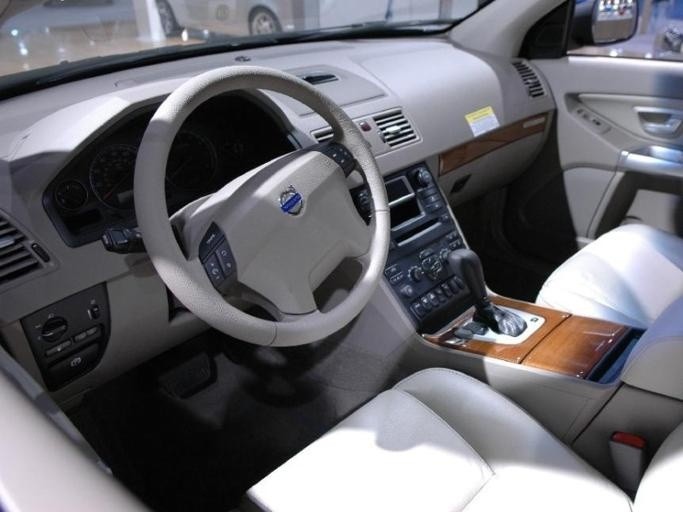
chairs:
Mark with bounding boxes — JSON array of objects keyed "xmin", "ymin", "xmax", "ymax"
[
  {"xmin": 532, "ymin": 222, "xmax": 683, "ymax": 398},
  {"xmin": 243, "ymin": 364, "xmax": 683, "ymax": 512}
]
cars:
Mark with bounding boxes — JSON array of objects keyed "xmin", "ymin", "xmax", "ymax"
[{"xmin": 158, "ymin": 2, "xmax": 296, "ymax": 44}]
